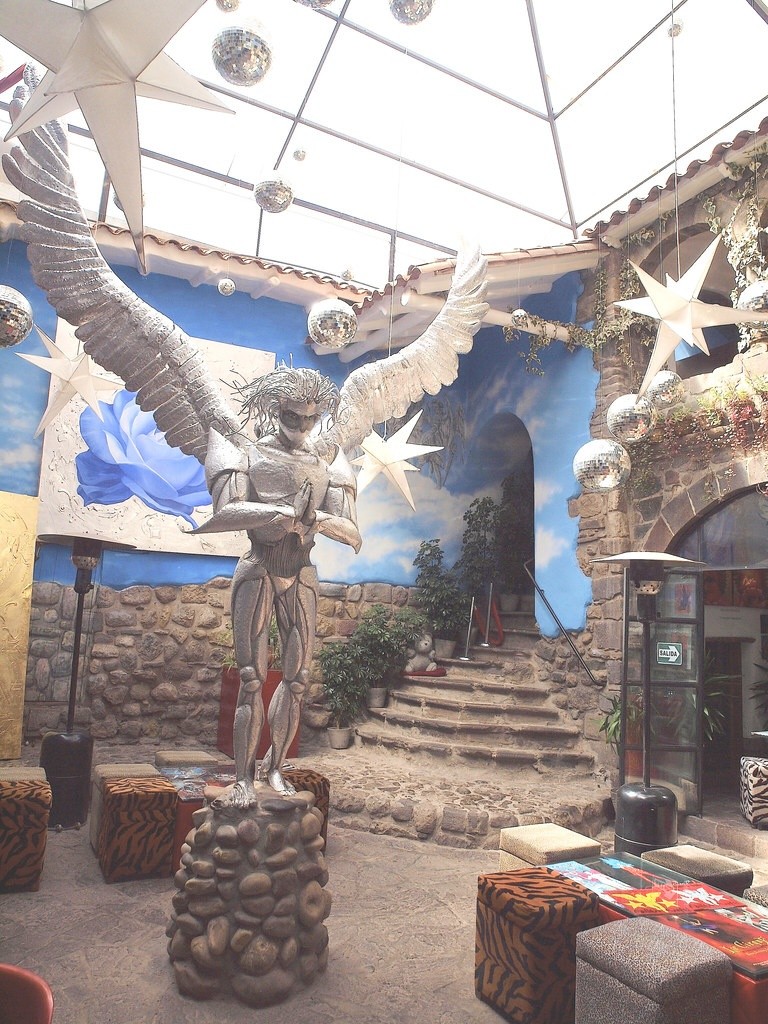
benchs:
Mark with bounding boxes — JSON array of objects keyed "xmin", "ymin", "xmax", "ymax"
[{"xmin": 545, "ymin": 850, "xmax": 768, "ymax": 1024}]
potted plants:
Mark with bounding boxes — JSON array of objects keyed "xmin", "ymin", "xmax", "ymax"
[
  {"xmin": 599, "ymin": 685, "xmax": 663, "ymax": 776},
  {"xmin": 663, "ymin": 647, "xmax": 744, "ymax": 779},
  {"xmin": 319, "ymin": 640, "xmax": 374, "ymax": 749},
  {"xmin": 217, "ymin": 605, "xmax": 300, "ymax": 760},
  {"xmin": 349, "ymin": 602, "xmax": 429, "ymax": 708},
  {"xmin": 451, "ymin": 496, "xmax": 498, "ymax": 645},
  {"xmin": 413, "ymin": 538, "xmax": 468, "ymax": 658},
  {"xmin": 498, "ymin": 470, "xmax": 534, "ymax": 613}
]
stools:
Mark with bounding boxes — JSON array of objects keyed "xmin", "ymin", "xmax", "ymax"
[
  {"xmin": 0, "ymin": 780, "xmax": 52, "ymax": 892},
  {"xmin": 739, "ymin": 757, "xmax": 768, "ymax": 831},
  {"xmin": 88, "ymin": 764, "xmax": 161, "ymax": 858},
  {"xmin": 98, "ymin": 777, "xmax": 178, "ymax": 884},
  {"xmin": 742, "ymin": 884, "xmax": 768, "ymax": 908},
  {"xmin": 574, "ymin": 916, "xmax": 733, "ymax": 1024},
  {"xmin": 0, "ymin": 767, "xmax": 46, "ymax": 780},
  {"xmin": 641, "ymin": 845, "xmax": 754, "ymax": 898},
  {"xmin": 155, "ymin": 751, "xmax": 330, "ymax": 878},
  {"xmin": 474, "ymin": 864, "xmax": 600, "ymax": 1024},
  {"xmin": 498, "ymin": 822, "xmax": 601, "ymax": 872}
]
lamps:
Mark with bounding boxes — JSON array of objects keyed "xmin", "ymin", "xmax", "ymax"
[
  {"xmin": 37, "ymin": 525, "xmax": 137, "ymax": 833},
  {"xmin": 589, "ymin": 552, "xmax": 708, "ymax": 857}
]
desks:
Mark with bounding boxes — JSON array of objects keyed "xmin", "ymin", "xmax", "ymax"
[{"xmin": 750, "ymin": 731, "xmax": 768, "ymax": 736}]
